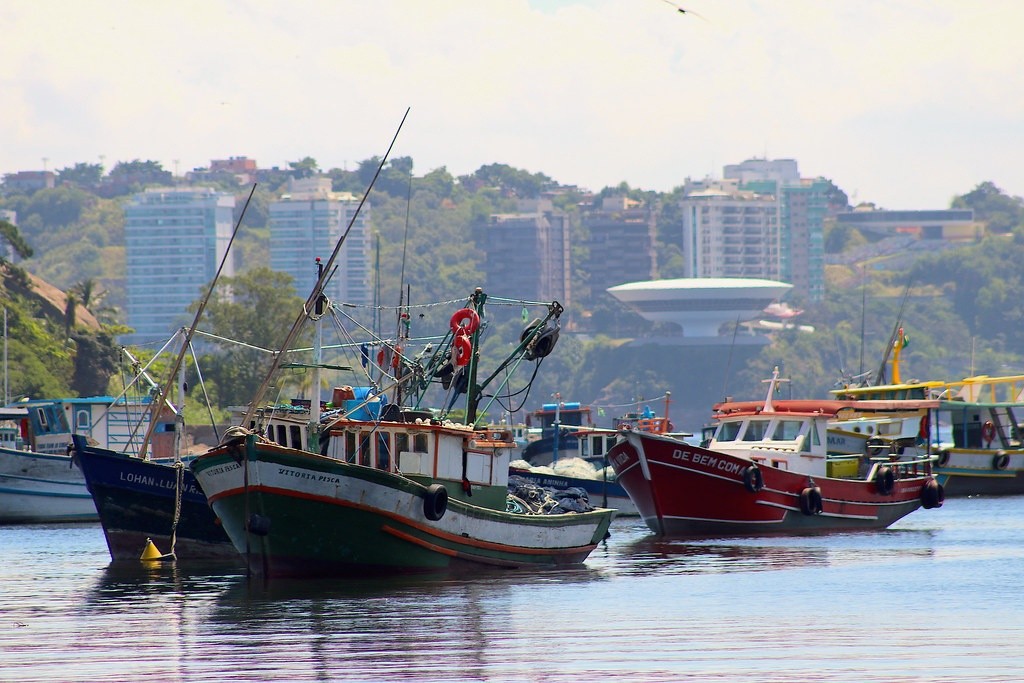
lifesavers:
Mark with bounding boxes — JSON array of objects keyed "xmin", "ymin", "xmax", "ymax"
[
  {"xmin": 376, "ymin": 345, "xmax": 401, "ymax": 368},
  {"xmin": 449, "ymin": 308, "xmax": 479, "ymax": 335},
  {"xmin": 669, "ymin": 423, "xmax": 673, "ymax": 431},
  {"xmin": 933, "ymin": 448, "xmax": 950, "ymax": 468},
  {"xmin": 801, "ymin": 487, "xmax": 823, "ymax": 516},
  {"xmin": 982, "ymin": 422, "xmax": 996, "ymax": 441},
  {"xmin": 865, "ymin": 435, "xmax": 884, "ymax": 456},
  {"xmin": 744, "ymin": 466, "xmax": 764, "ymax": 493},
  {"xmin": 423, "ymin": 483, "xmax": 448, "ymax": 521},
  {"xmin": 877, "ymin": 467, "xmax": 894, "ymax": 495},
  {"xmin": 451, "ymin": 336, "xmax": 472, "ymax": 366},
  {"xmin": 993, "ymin": 451, "xmax": 1010, "ymax": 470},
  {"xmin": 920, "ymin": 478, "xmax": 944, "ymax": 510},
  {"xmin": 919, "ymin": 415, "xmax": 928, "ymax": 440}
]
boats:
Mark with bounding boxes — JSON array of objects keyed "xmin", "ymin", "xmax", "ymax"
[
  {"xmin": 65, "ymin": 183, "xmax": 341, "ymax": 563},
  {"xmin": 0, "ymin": 397, "xmax": 200, "ymax": 525},
  {"xmin": 186, "ymin": 105, "xmax": 619, "ymax": 580},
  {"xmin": 506, "ymin": 392, "xmax": 694, "ymax": 518},
  {"xmin": 607, "ymin": 364, "xmax": 946, "ymax": 538},
  {"xmin": 826, "ymin": 266, "xmax": 1024, "ymax": 498}
]
